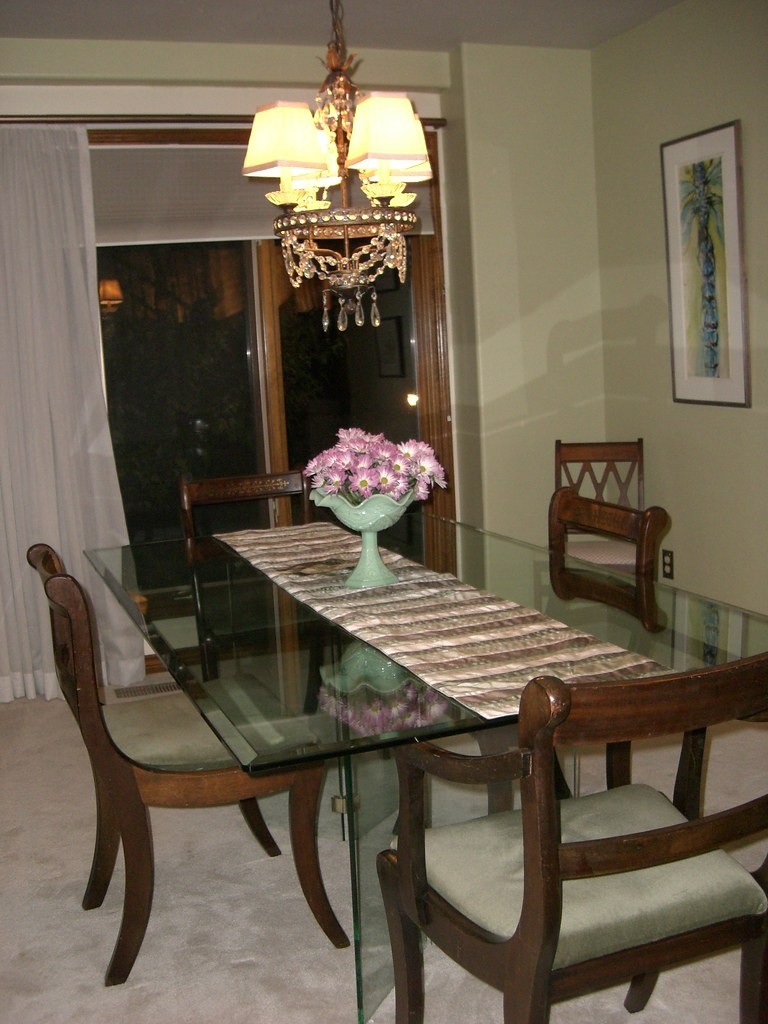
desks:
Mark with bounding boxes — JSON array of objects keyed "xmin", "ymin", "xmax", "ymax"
[{"xmin": 83, "ymin": 508, "xmax": 768, "ymax": 1024}]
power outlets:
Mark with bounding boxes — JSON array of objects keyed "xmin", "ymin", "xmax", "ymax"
[{"xmin": 662, "ymin": 549, "xmax": 674, "ymax": 579}]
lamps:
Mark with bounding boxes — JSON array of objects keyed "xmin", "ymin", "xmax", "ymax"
[
  {"xmin": 98, "ymin": 276, "xmax": 124, "ymax": 313},
  {"xmin": 239, "ymin": 0, "xmax": 433, "ymax": 332}
]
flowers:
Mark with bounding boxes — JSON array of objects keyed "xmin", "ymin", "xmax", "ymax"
[
  {"xmin": 318, "ymin": 688, "xmax": 450, "ymax": 736},
  {"xmin": 302, "ymin": 425, "xmax": 447, "ymax": 505}
]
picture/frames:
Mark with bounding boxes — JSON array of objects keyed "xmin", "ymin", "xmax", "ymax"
[
  {"xmin": 373, "ymin": 315, "xmax": 404, "ymax": 379},
  {"xmin": 371, "ymin": 260, "xmax": 402, "ymax": 293},
  {"xmin": 659, "ymin": 117, "xmax": 752, "ymax": 406}
]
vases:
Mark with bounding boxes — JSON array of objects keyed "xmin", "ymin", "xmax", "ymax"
[
  {"xmin": 320, "ymin": 639, "xmax": 422, "ymax": 695},
  {"xmin": 308, "ymin": 487, "xmax": 417, "ymax": 588}
]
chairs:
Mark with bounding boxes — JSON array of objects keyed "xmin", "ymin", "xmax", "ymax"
[
  {"xmin": 179, "ymin": 466, "xmax": 311, "ymax": 537},
  {"xmin": 26, "ymin": 544, "xmax": 351, "ymax": 986},
  {"xmin": 479, "ymin": 437, "xmax": 667, "ymax": 799},
  {"xmin": 374, "ymin": 650, "xmax": 768, "ymax": 1024}
]
